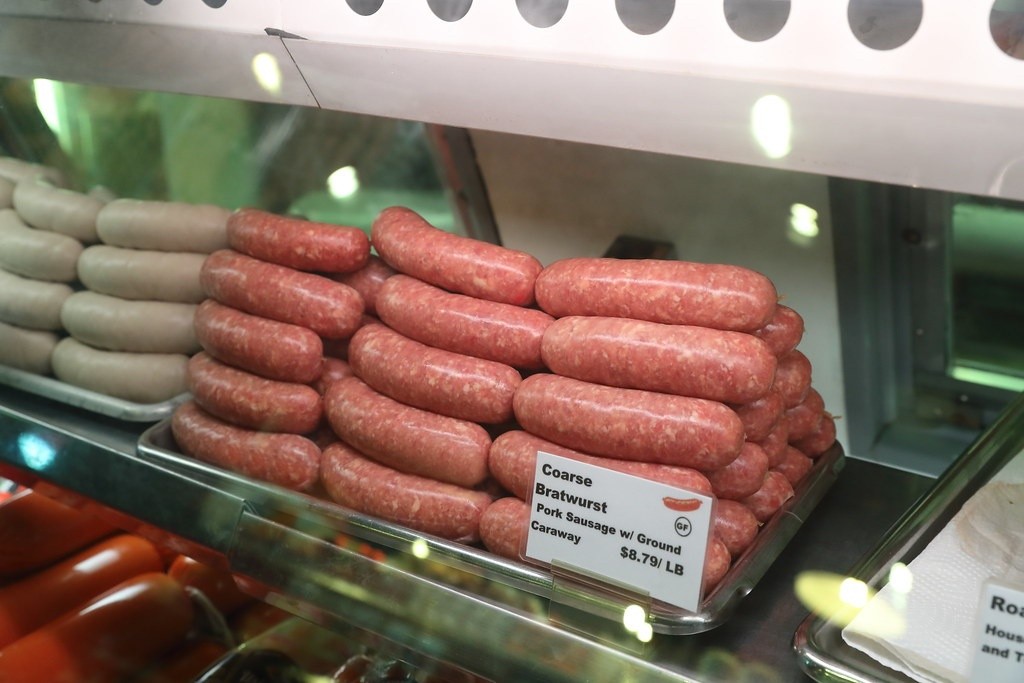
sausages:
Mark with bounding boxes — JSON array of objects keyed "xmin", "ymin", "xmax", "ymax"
[
  {"xmin": 0, "ymin": 476, "xmax": 270, "ymax": 683},
  {"xmin": 0, "ymin": 156, "xmax": 234, "ymax": 406},
  {"xmin": 168, "ymin": 205, "xmax": 838, "ymax": 601}
]
